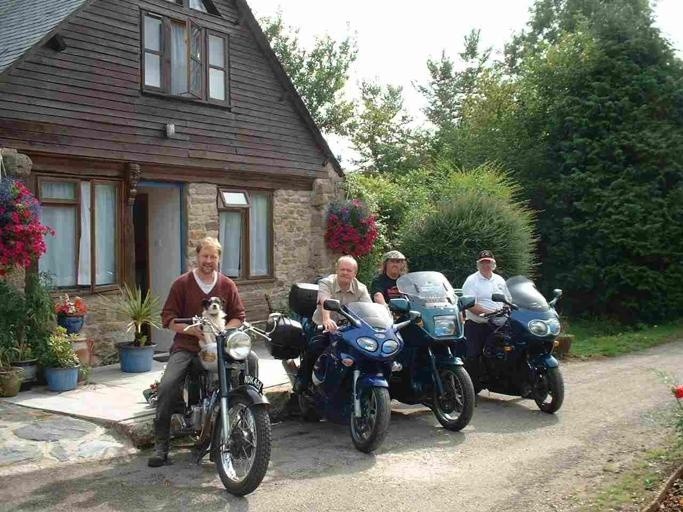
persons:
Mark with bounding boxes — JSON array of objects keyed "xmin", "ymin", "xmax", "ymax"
[
  {"xmin": 148, "ymin": 237, "xmax": 259, "ymax": 468},
  {"xmin": 462, "ymin": 251, "xmax": 511, "ymax": 409},
  {"xmin": 284, "ymin": 255, "xmax": 373, "ymax": 408},
  {"xmin": 371, "ymin": 251, "xmax": 409, "ymax": 320}
]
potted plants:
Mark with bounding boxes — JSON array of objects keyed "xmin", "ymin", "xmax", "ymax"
[
  {"xmin": 97, "ymin": 285, "xmax": 162, "ymax": 373},
  {"xmin": 43, "ymin": 326, "xmax": 81, "ymax": 391},
  {"xmin": 0, "ymin": 346, "xmax": 24, "ymax": 397},
  {"xmin": 0, "ymin": 273, "xmax": 54, "ymax": 391}
]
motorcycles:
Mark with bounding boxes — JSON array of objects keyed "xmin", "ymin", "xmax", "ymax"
[
  {"xmin": 149, "ymin": 312, "xmax": 285, "ymax": 497},
  {"xmin": 452, "ymin": 276, "xmax": 564, "ymax": 415},
  {"xmin": 389, "ymin": 269, "xmax": 475, "ymax": 433},
  {"xmin": 267, "ymin": 282, "xmax": 421, "ymax": 453}
]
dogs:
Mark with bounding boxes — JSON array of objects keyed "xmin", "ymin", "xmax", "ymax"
[{"xmin": 197, "ymin": 296, "xmax": 227, "ymax": 371}]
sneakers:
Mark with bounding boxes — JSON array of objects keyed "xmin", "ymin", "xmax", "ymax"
[{"xmin": 147, "ymin": 449, "xmax": 168, "ymax": 467}]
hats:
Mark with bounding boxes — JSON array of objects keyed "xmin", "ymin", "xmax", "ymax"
[
  {"xmin": 479, "ymin": 250, "xmax": 494, "ymax": 261},
  {"xmin": 385, "ymin": 251, "xmax": 405, "ymax": 262}
]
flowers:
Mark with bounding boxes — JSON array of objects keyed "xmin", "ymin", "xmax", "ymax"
[
  {"xmin": 54, "ymin": 293, "xmax": 89, "ymax": 315},
  {"xmin": 57, "ymin": 316, "xmax": 83, "ymax": 335},
  {"xmin": 325, "ymin": 198, "xmax": 379, "ymax": 258},
  {"xmin": 0, "ymin": 178, "xmax": 56, "ymax": 271}
]
